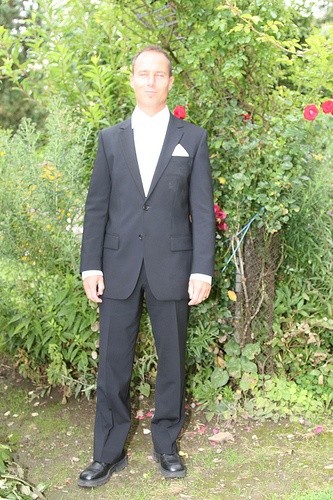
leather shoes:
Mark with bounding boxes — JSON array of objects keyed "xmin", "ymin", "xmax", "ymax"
[
  {"xmin": 152, "ymin": 452, "xmax": 186, "ymax": 477},
  {"xmin": 78, "ymin": 448, "xmax": 128, "ymax": 487}
]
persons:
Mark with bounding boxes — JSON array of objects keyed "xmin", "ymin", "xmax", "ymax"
[{"xmin": 76, "ymin": 47, "xmax": 216, "ymax": 486}]
